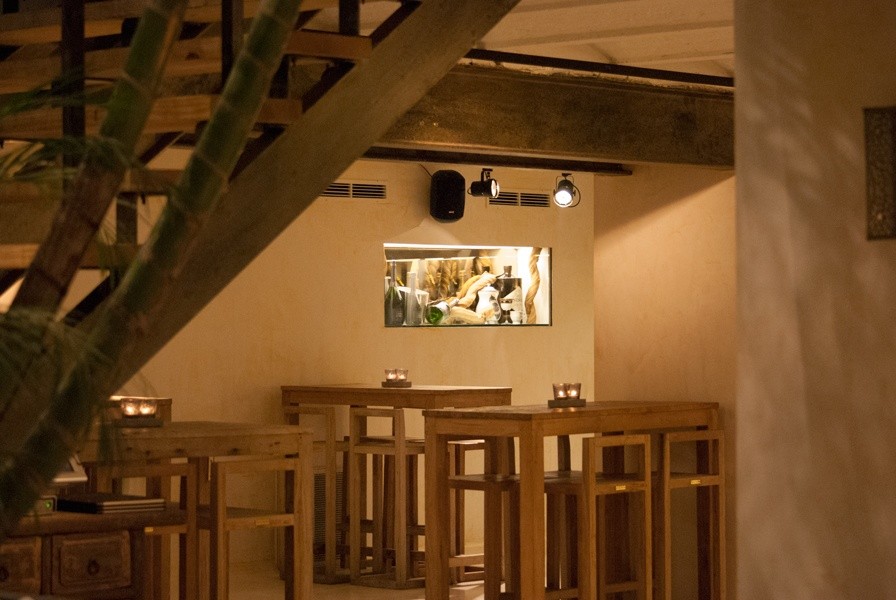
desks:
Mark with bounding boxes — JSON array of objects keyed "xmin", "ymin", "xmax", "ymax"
[
  {"xmin": 282, "ymin": 385, "xmax": 512, "ymax": 600},
  {"xmin": 422, "ymin": 400, "xmax": 719, "ymax": 600},
  {"xmin": 78, "ymin": 420, "xmax": 315, "ymax": 600}
]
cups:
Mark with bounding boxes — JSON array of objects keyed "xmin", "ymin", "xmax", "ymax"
[
  {"xmin": 553, "ymin": 382, "xmax": 582, "ymax": 400},
  {"xmin": 509, "ymin": 311, "xmax": 522, "ymax": 324},
  {"xmin": 120, "ymin": 398, "xmax": 157, "ymax": 417},
  {"xmin": 385, "ymin": 369, "xmax": 409, "ymax": 382}
]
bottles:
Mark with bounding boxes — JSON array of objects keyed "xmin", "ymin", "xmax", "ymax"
[{"xmin": 384, "ymin": 262, "xmax": 459, "ymax": 324}]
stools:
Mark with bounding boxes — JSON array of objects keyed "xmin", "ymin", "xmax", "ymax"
[{"xmin": 92, "ymin": 407, "xmax": 728, "ymax": 600}]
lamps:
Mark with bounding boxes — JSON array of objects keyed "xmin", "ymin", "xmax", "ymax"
[
  {"xmin": 553, "ymin": 173, "xmax": 581, "ymax": 208},
  {"xmin": 467, "ymin": 168, "xmax": 500, "ymax": 198}
]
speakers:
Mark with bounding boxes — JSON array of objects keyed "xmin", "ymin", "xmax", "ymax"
[{"xmin": 431, "ymin": 170, "xmax": 465, "ymax": 219}]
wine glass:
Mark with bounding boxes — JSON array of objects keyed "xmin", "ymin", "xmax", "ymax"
[{"xmin": 500, "ymin": 298, "xmax": 513, "ymax": 324}]
